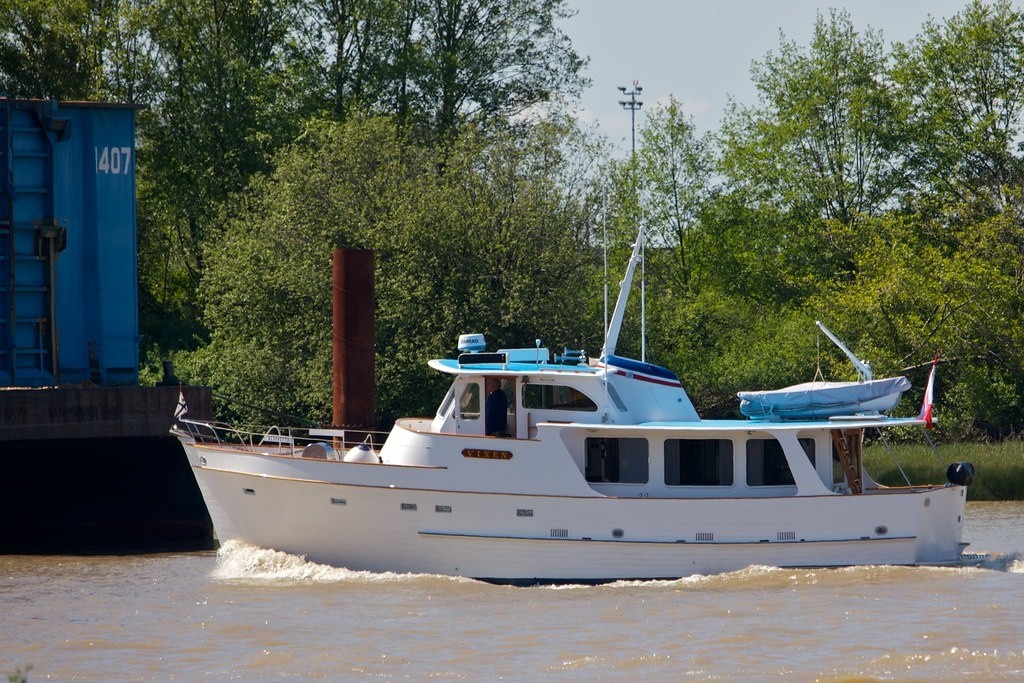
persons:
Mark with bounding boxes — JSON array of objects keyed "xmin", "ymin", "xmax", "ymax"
[{"xmin": 485, "ymin": 376, "xmax": 508, "ymax": 437}]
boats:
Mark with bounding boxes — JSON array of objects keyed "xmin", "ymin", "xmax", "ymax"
[
  {"xmin": 726, "ymin": 322, "xmax": 912, "ymax": 420},
  {"xmin": 165, "ymin": 187, "xmax": 981, "ymax": 589}
]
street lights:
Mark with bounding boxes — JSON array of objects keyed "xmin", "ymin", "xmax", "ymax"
[{"xmin": 618, "ymin": 79, "xmax": 643, "ymax": 182}]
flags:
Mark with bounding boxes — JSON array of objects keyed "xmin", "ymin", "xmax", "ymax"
[{"xmin": 917, "ymin": 354, "xmax": 938, "ymax": 429}]
